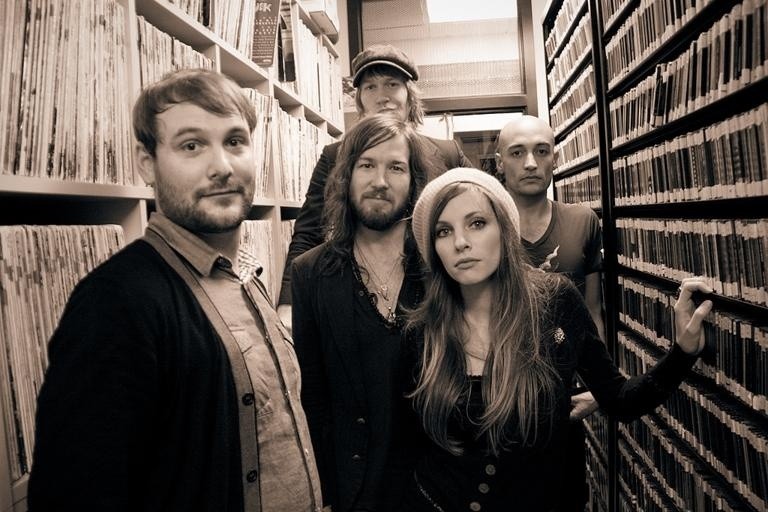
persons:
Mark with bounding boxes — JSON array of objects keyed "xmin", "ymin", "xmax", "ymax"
[
  {"xmin": 396, "ymin": 165, "xmax": 713, "ymax": 512},
  {"xmin": 290, "ymin": 111, "xmax": 437, "ymax": 510},
  {"xmin": 491, "ymin": 113, "xmax": 607, "ymax": 512},
  {"xmin": 275, "ymin": 42, "xmax": 481, "ymax": 341}
]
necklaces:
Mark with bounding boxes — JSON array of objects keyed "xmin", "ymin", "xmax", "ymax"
[
  {"xmin": 356, "ymin": 243, "xmax": 398, "ymax": 324},
  {"xmin": 465, "ymin": 348, "xmax": 489, "ymax": 364},
  {"xmin": 354, "ymin": 238, "xmax": 401, "ymax": 301}
]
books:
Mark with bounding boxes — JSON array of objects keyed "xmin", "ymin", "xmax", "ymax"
[
  {"xmin": 2, "ymin": 3, "xmax": 348, "ymax": 205},
  {"xmin": 2, "ymin": 218, "xmax": 298, "ymax": 481},
  {"xmin": 545, "ymin": 1, "xmax": 767, "ymax": 208},
  {"xmin": 576, "ymin": 217, "xmax": 768, "ymax": 509}
]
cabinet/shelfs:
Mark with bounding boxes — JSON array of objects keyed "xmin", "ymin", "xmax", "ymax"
[
  {"xmin": 539, "ymin": 0, "xmax": 768, "ymax": 512},
  {"xmin": 1, "ymin": 0, "xmax": 348, "ymax": 510}
]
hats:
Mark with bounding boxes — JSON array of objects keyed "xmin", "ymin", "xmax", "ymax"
[
  {"xmin": 351, "ymin": 45, "xmax": 418, "ymax": 87},
  {"xmin": 411, "ymin": 166, "xmax": 520, "ymax": 271}
]
